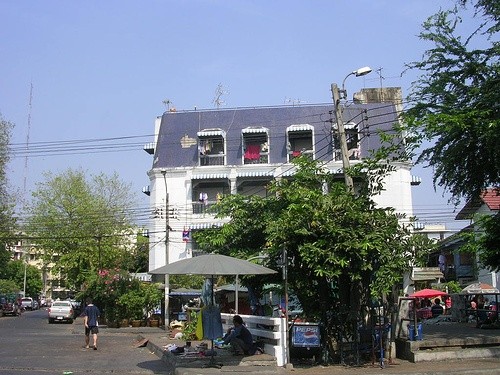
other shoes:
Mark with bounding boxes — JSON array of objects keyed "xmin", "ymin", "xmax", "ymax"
[
  {"xmin": 92, "ymin": 345, "xmax": 97, "ymax": 350},
  {"xmin": 232, "ymin": 351, "xmax": 245, "ymax": 356},
  {"xmin": 164, "ymin": 341, "xmax": 195, "ymax": 357},
  {"xmin": 82, "ymin": 344, "xmax": 89, "ymax": 349}
]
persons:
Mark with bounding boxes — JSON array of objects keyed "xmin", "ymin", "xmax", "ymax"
[
  {"xmin": 224, "ymin": 316, "xmax": 253, "ymax": 354},
  {"xmin": 83, "ymin": 298, "xmax": 101, "ymax": 350},
  {"xmin": 404, "ymin": 294, "xmax": 496, "ymax": 320}
]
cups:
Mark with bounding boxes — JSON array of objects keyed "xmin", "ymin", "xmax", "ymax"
[{"xmin": 186, "ymin": 342, "xmax": 191, "ymax": 346}]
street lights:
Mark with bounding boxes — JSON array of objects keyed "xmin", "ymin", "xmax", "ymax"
[
  {"xmin": 160, "ymin": 169, "xmax": 170, "ymax": 330},
  {"xmin": 330, "ymin": 66, "xmax": 372, "ymax": 192}
]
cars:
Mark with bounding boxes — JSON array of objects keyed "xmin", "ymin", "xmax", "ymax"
[
  {"xmin": 48, "ymin": 298, "xmax": 80, "ymax": 323},
  {"xmin": 20, "ymin": 296, "xmax": 52, "ymax": 311}
]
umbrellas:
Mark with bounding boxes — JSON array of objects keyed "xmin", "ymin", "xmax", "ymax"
[
  {"xmin": 409, "ymin": 288, "xmax": 449, "ymax": 300},
  {"xmin": 461, "ymin": 282, "xmax": 500, "ymax": 294},
  {"xmin": 148, "ymin": 252, "xmax": 278, "ymax": 369}
]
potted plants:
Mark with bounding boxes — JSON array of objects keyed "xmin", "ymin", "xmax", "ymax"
[{"xmin": 98, "ymin": 283, "xmax": 164, "ymax": 328}]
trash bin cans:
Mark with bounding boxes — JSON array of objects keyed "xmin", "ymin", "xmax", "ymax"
[{"xmin": 408, "ymin": 324, "xmax": 423, "ymax": 341}]
